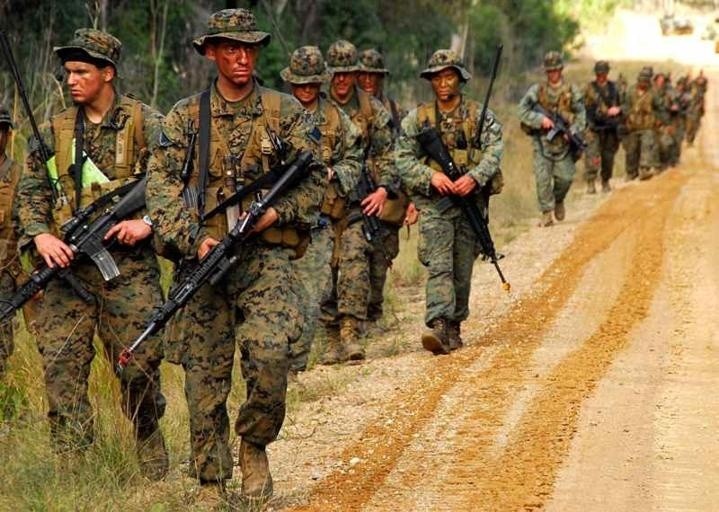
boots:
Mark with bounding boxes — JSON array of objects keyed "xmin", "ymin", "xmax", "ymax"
[
  {"xmin": 537, "ymin": 211, "xmax": 552, "ymax": 227},
  {"xmin": 136, "ymin": 428, "xmax": 169, "ymax": 480},
  {"xmin": 317, "ymin": 317, "xmax": 381, "ymax": 364},
  {"xmin": 238, "ymin": 437, "xmax": 273, "ymax": 503},
  {"xmin": 422, "ymin": 316, "xmax": 463, "ymax": 355},
  {"xmin": 587, "ymin": 180, "xmax": 595, "ymax": 193},
  {"xmin": 602, "ymin": 179, "xmax": 610, "ymax": 191},
  {"xmin": 554, "ymin": 197, "xmax": 565, "ymax": 220}
]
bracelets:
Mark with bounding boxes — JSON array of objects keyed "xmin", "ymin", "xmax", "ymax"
[{"xmin": 140, "ymin": 214, "xmax": 152, "ymax": 231}]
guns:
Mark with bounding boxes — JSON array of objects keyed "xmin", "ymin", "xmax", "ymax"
[
  {"xmin": 1, "ymin": 175, "xmax": 148, "ymax": 324},
  {"xmin": 412, "ymin": 127, "xmax": 507, "ymax": 285},
  {"xmin": 118, "ymin": 149, "xmax": 313, "ymax": 372},
  {"xmin": 532, "ymin": 101, "xmax": 588, "ymax": 151},
  {"xmin": 471, "ymin": 43, "xmax": 504, "ymax": 151},
  {"xmin": 347, "ymin": 164, "xmax": 395, "ymax": 268},
  {"xmin": 611, "ymin": 75, "xmax": 623, "ymax": 131}
]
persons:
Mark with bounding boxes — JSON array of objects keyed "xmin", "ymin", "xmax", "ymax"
[
  {"xmin": 144, "ymin": 7, "xmax": 322, "ymax": 505},
  {"xmin": 19, "ymin": 25, "xmax": 168, "ymax": 482},
  {"xmin": 0, "ymin": 106, "xmax": 48, "ymax": 375},
  {"xmin": 517, "ymin": 49, "xmax": 710, "ymax": 229},
  {"xmin": 313, "ymin": 39, "xmax": 396, "ymax": 366},
  {"xmin": 392, "ymin": 47, "xmax": 504, "ymax": 356},
  {"xmin": 278, "ymin": 45, "xmax": 366, "ymax": 381},
  {"xmin": 352, "ymin": 47, "xmax": 419, "ymax": 337}
]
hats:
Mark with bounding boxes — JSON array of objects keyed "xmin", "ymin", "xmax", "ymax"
[
  {"xmin": 193, "ymin": 8, "xmax": 271, "ymax": 56},
  {"xmin": 53, "ymin": 27, "xmax": 122, "ymax": 78},
  {"xmin": 543, "ymin": 50, "xmax": 563, "ymax": 71},
  {"xmin": 595, "ymin": 61, "xmax": 609, "ymax": 72},
  {"xmin": 420, "ymin": 48, "xmax": 471, "ymax": 83},
  {"xmin": 279, "ymin": 41, "xmax": 390, "ymax": 86}
]
